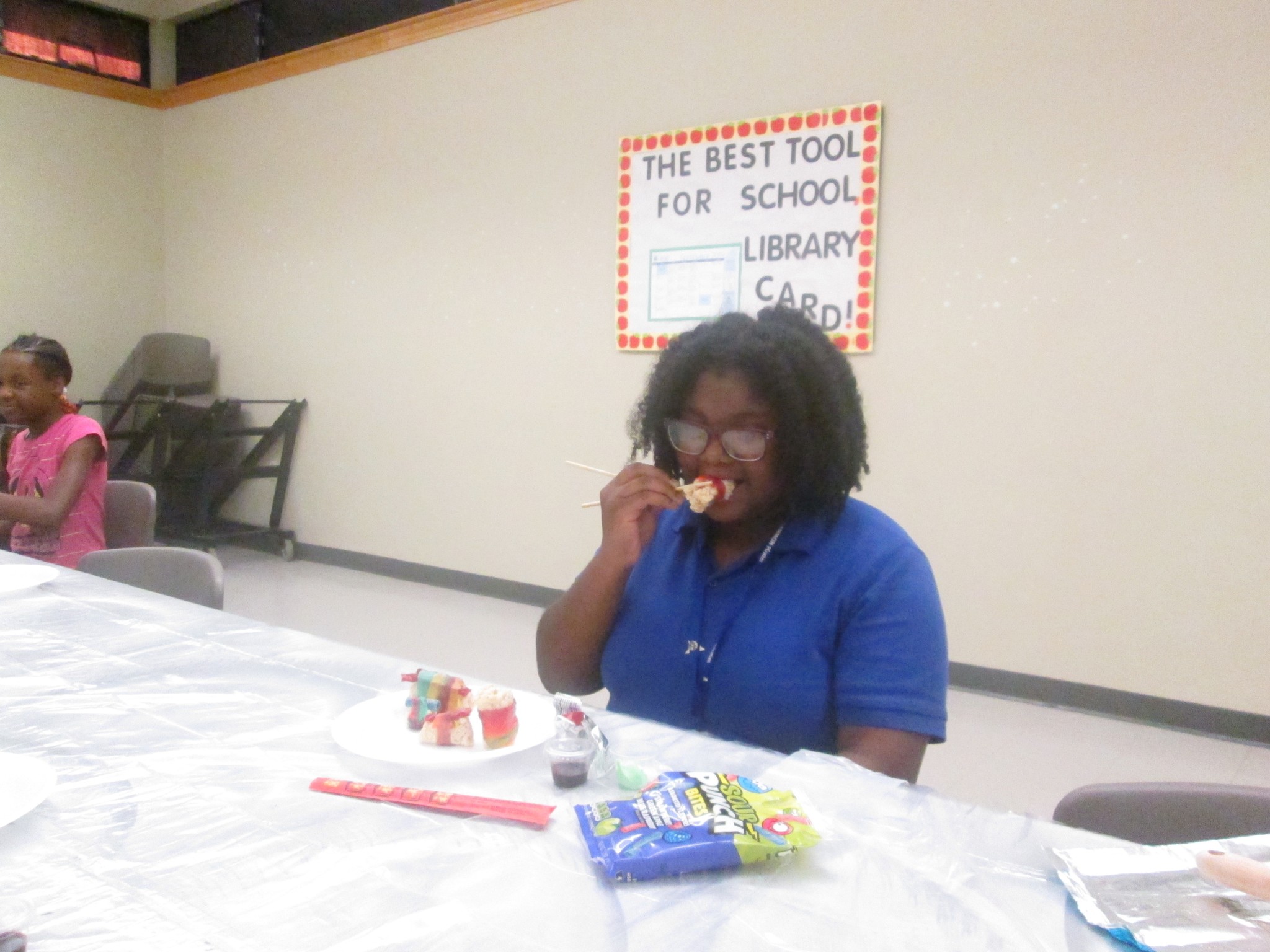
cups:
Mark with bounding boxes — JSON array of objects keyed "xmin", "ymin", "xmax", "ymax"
[
  {"xmin": 545, "ymin": 737, "xmax": 597, "ymax": 788},
  {"xmin": 608, "ymin": 740, "xmax": 654, "ymax": 790}
]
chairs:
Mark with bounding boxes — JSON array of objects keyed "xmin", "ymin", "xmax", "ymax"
[
  {"xmin": 103, "ymin": 480, "xmax": 156, "ymax": 548},
  {"xmin": 78, "ymin": 547, "xmax": 225, "ymax": 610},
  {"xmin": 1054, "ymin": 781, "xmax": 1270, "ymax": 848}
]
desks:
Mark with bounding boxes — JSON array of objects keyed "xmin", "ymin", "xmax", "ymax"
[{"xmin": 0, "ymin": 550, "xmax": 1270, "ymax": 952}]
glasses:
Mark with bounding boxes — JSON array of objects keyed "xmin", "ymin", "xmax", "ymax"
[{"xmin": 662, "ymin": 419, "xmax": 781, "ymax": 462}]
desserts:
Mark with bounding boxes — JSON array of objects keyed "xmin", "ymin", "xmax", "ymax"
[
  {"xmin": 683, "ymin": 475, "xmax": 736, "ymax": 513},
  {"xmin": 400, "ymin": 669, "xmax": 520, "ymax": 749}
]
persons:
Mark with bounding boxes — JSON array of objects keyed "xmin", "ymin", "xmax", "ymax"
[
  {"xmin": 0, "ymin": 333, "xmax": 109, "ymax": 570},
  {"xmin": 535, "ymin": 305, "xmax": 950, "ymax": 785}
]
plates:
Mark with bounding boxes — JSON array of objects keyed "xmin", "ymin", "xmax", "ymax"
[{"xmin": 332, "ymin": 687, "xmax": 558, "ymax": 764}]
